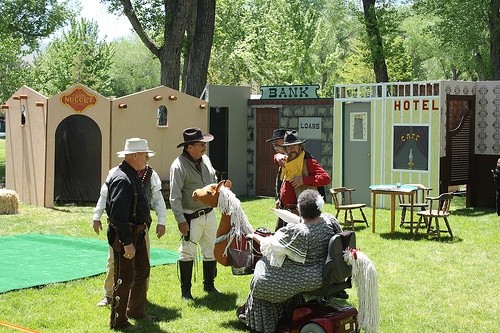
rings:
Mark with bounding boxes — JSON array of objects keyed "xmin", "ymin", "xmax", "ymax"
[{"xmin": 127, "ymin": 254, "xmax": 131, "ymax": 257}]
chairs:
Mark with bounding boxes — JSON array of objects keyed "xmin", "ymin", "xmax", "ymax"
[
  {"xmin": 398, "ymin": 184, "xmax": 434, "ymax": 230},
  {"xmin": 298, "ymin": 231, "xmax": 357, "ymax": 305},
  {"xmin": 329, "ymin": 187, "xmax": 369, "ymax": 231},
  {"xmin": 413, "ymin": 192, "xmax": 454, "ymax": 240}
]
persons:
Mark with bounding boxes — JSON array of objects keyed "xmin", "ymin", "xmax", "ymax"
[
  {"xmin": 239, "ymin": 189, "xmax": 343, "ymax": 333},
  {"xmin": 266, "ymin": 128, "xmax": 332, "ymax": 234},
  {"xmin": 169, "ymin": 127, "xmax": 225, "ymax": 301},
  {"xmin": 92, "ymin": 138, "xmax": 167, "ymax": 333}
]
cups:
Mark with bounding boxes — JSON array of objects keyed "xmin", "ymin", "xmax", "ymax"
[{"xmin": 397, "ymin": 183, "xmax": 401, "ymax": 189}]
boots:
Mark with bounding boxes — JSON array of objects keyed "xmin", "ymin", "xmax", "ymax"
[
  {"xmin": 109, "ymin": 287, "xmax": 143, "ymax": 331},
  {"xmin": 127, "ymin": 289, "xmax": 158, "ymax": 322},
  {"xmin": 202, "ymin": 260, "xmax": 220, "ymax": 294},
  {"xmin": 176, "ymin": 260, "xmax": 195, "ymax": 300}
]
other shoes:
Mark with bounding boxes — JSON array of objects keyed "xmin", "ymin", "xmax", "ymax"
[{"xmin": 95, "ymin": 297, "xmax": 112, "ymax": 307}]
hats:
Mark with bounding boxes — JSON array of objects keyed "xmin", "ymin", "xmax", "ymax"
[
  {"xmin": 116, "ymin": 138, "xmax": 156, "ymax": 158},
  {"xmin": 266, "ymin": 129, "xmax": 292, "ymax": 143},
  {"xmin": 274, "ymin": 130, "xmax": 310, "ymax": 147},
  {"xmin": 177, "ymin": 127, "xmax": 214, "ymax": 149}
]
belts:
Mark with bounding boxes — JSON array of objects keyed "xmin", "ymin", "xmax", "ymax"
[{"xmin": 183, "ymin": 208, "xmax": 213, "ymax": 220}]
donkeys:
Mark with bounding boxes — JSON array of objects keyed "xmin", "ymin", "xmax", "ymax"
[{"xmin": 191, "ymin": 179, "xmax": 276, "ymax": 267}]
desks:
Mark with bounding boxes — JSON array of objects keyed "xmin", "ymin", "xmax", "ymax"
[{"xmin": 368, "ymin": 184, "xmax": 415, "ymax": 241}]
